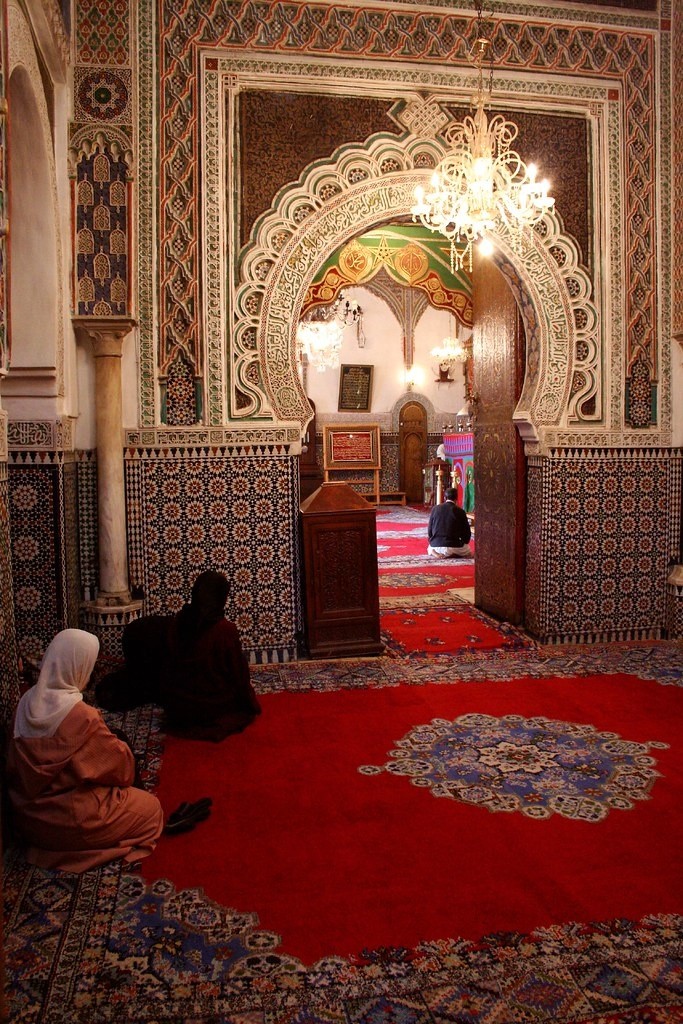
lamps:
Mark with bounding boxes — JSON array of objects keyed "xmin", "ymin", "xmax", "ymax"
[
  {"xmin": 410, "ymin": 0, "xmax": 555, "ymax": 274},
  {"xmin": 428, "ymin": 311, "xmax": 471, "ymax": 371},
  {"xmin": 296, "ymin": 290, "xmax": 366, "ymax": 373}
]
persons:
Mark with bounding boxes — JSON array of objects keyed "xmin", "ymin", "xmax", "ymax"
[
  {"xmin": 154, "ymin": 572, "xmax": 262, "ymax": 743},
  {"xmin": 13, "ymin": 629, "xmax": 164, "ymax": 875},
  {"xmin": 428, "ymin": 488, "xmax": 474, "ymax": 558}
]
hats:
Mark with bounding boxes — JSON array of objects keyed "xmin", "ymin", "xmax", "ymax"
[{"xmin": 445, "ymin": 488, "xmax": 457, "ymax": 500}]
